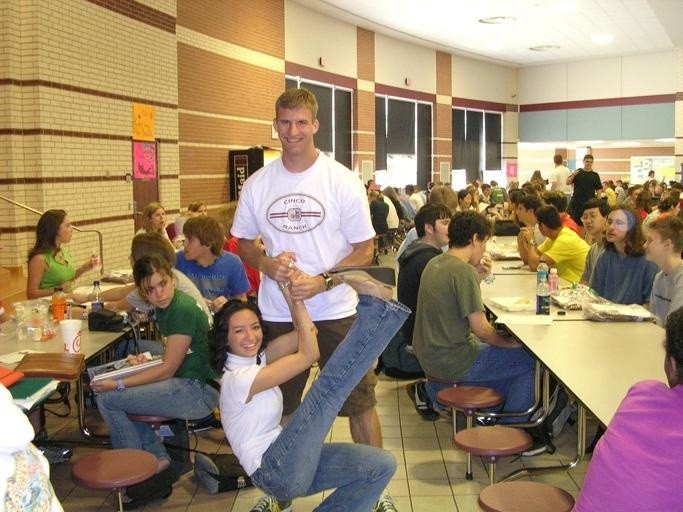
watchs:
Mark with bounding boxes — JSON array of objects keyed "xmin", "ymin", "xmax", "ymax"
[{"xmin": 319, "ymin": 271, "xmax": 334, "ymax": 291}]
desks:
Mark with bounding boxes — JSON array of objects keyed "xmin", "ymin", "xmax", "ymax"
[{"xmin": 0, "ymin": 269, "xmax": 152, "ymax": 445}]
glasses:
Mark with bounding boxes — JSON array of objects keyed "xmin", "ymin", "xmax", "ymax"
[{"xmin": 579, "ymin": 212, "xmax": 603, "ymax": 223}]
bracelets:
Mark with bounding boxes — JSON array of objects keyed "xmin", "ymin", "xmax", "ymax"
[
  {"xmin": 278, "ymin": 279, "xmax": 291, "ymax": 289},
  {"xmin": 117, "ymin": 378, "xmax": 128, "ymax": 392}
]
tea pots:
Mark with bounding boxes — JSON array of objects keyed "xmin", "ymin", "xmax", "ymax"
[{"xmin": 25, "ymin": 301, "xmax": 55, "ymax": 341}]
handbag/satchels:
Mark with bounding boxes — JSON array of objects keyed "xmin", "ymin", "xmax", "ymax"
[
  {"xmin": 15, "ymin": 352, "xmax": 86, "ymax": 383},
  {"xmin": 89, "ymin": 309, "xmax": 138, "ymax": 358},
  {"xmin": 186, "ymin": 449, "xmax": 255, "ymax": 494}
]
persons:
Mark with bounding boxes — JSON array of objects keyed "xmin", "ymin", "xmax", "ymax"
[
  {"xmin": 227, "ymin": 84, "xmax": 400, "ymax": 511},
  {"xmin": 404, "ymin": 209, "xmax": 550, "ymax": 451},
  {"xmin": 207, "ymin": 250, "xmax": 412, "ymax": 511},
  {"xmin": 371, "ymin": 151, "xmax": 683, "ymax": 309},
  {"xmin": 86, "ymin": 250, "xmax": 221, "ymax": 505},
  {"xmin": 67, "ymin": 205, "xmax": 259, "ymax": 383},
  {"xmin": 1, "ymin": 384, "xmax": 69, "ymax": 512},
  {"xmin": 25, "ymin": 210, "xmax": 81, "ymax": 295},
  {"xmin": 568, "ymin": 306, "xmax": 682, "ymax": 512},
  {"xmin": 395, "ymin": 200, "xmax": 453, "ymax": 422}
]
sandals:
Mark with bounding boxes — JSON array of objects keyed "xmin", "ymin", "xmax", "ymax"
[{"xmin": 329, "ymin": 264, "xmax": 397, "ymax": 303}]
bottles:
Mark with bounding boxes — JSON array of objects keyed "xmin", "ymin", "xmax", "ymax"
[
  {"xmin": 534, "ymin": 278, "xmax": 551, "ymax": 316},
  {"xmin": 90, "ymin": 251, "xmax": 100, "ymax": 270},
  {"xmin": 51, "ymin": 286, "xmax": 65, "ymax": 321},
  {"xmin": 548, "ymin": 267, "xmax": 561, "ymax": 295},
  {"xmin": 535, "ymin": 258, "xmax": 548, "ymax": 284},
  {"xmin": 90, "ymin": 280, "xmax": 103, "ymax": 312}
]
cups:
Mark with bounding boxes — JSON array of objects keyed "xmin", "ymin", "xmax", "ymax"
[{"xmin": 58, "ymin": 319, "xmax": 81, "ymax": 354}]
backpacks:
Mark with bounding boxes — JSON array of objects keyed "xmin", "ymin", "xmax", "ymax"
[{"xmin": 526, "ymin": 379, "xmax": 576, "ymax": 454}]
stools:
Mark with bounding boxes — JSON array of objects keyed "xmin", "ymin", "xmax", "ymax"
[
  {"xmin": 70, "ymin": 447, "xmax": 162, "ymax": 512},
  {"xmin": 477, "ymin": 482, "xmax": 573, "ymax": 512},
  {"xmin": 128, "ymin": 414, "xmax": 173, "ymax": 441},
  {"xmin": 435, "ymin": 384, "xmax": 506, "ymax": 481},
  {"xmin": 370, "ymin": 220, "xmax": 415, "ymax": 267},
  {"xmin": 453, "ymin": 426, "xmax": 533, "ymax": 487}
]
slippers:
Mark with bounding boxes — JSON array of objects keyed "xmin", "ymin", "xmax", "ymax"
[{"xmin": 122, "ymin": 476, "xmax": 172, "ymax": 509}]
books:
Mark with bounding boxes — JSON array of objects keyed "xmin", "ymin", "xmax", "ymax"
[
  {"xmin": 88, "ymin": 350, "xmax": 164, "ymax": 382},
  {"xmin": 0, "ymin": 365, "xmax": 61, "ymax": 414}
]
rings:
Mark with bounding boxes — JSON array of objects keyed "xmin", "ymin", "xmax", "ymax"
[{"xmin": 288, "ymin": 262, "xmax": 293, "ymax": 268}]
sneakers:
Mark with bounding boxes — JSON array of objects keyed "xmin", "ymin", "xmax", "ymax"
[
  {"xmin": 521, "ymin": 437, "xmax": 548, "ymax": 458},
  {"xmin": 248, "ymin": 494, "xmax": 294, "ymax": 512},
  {"xmin": 374, "ymin": 488, "xmax": 398, "ymax": 512},
  {"xmin": 405, "ymin": 382, "xmax": 441, "ymax": 421}
]
